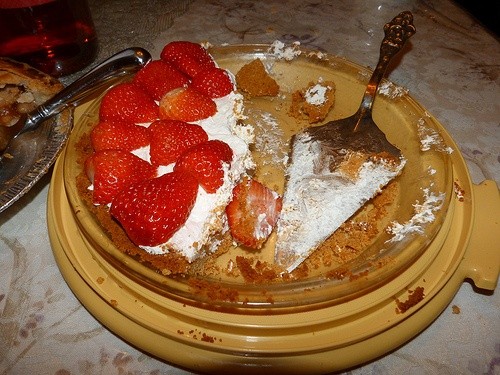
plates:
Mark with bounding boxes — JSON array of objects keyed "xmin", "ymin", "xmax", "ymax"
[{"xmin": 0, "ymin": 54, "xmax": 74, "ymax": 213}]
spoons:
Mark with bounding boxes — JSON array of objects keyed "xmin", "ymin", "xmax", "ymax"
[{"xmin": 0, "ymin": 47, "xmax": 151, "ymax": 154}]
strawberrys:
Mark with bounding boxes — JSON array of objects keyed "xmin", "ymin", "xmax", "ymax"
[{"xmin": 229, "ymin": 181, "xmax": 283, "ymax": 249}]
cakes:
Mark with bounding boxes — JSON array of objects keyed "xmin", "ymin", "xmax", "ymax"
[{"xmin": 85, "ymin": 41, "xmax": 258, "ymax": 262}]
